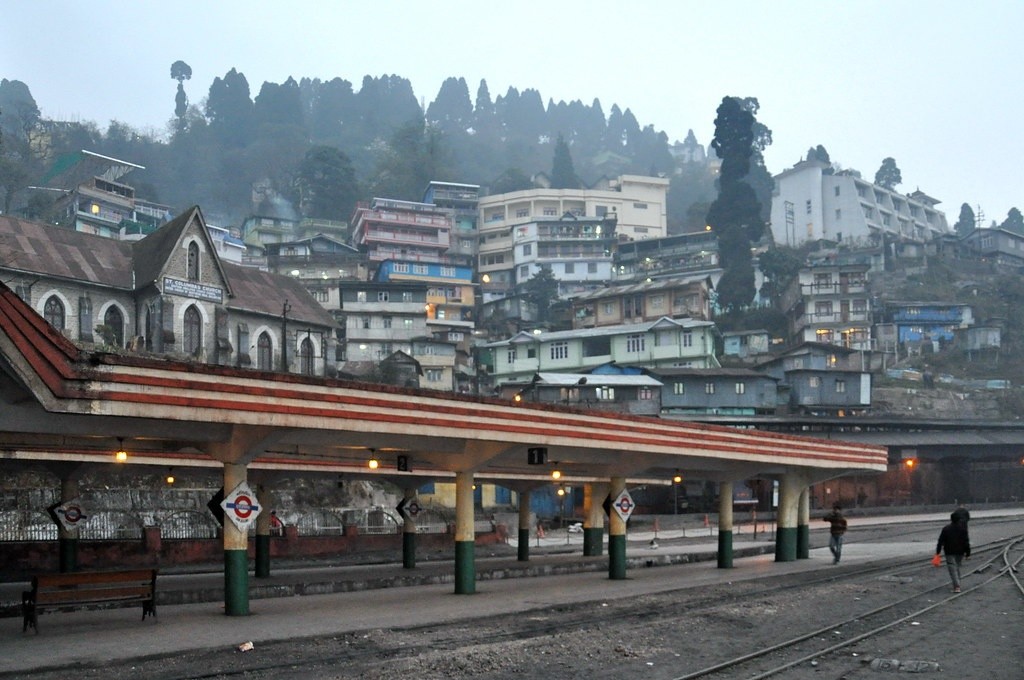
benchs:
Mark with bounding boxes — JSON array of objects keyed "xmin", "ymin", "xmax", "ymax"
[{"xmin": 21, "ymin": 565, "xmax": 160, "ymax": 635}]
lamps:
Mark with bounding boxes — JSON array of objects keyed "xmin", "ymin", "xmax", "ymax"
[
  {"xmin": 368, "ymin": 447, "xmax": 378, "ymax": 469},
  {"xmin": 167, "ymin": 466, "xmax": 174, "ymax": 484},
  {"xmin": 116, "ymin": 437, "xmax": 127, "ymax": 460},
  {"xmin": 673, "ymin": 469, "xmax": 682, "ymax": 482},
  {"xmin": 551, "ymin": 461, "xmax": 560, "ymax": 478}
]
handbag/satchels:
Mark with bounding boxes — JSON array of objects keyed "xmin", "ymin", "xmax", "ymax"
[{"xmin": 932, "ymin": 555, "xmax": 941, "ymax": 568}]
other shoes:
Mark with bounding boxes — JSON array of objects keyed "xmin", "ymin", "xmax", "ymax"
[{"xmin": 950, "ymin": 586, "xmax": 961, "ymax": 593}]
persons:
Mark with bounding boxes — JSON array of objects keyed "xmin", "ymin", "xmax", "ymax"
[
  {"xmin": 750, "ymin": 503, "xmax": 759, "ymax": 523},
  {"xmin": 936, "ymin": 507, "xmax": 971, "ymax": 592},
  {"xmin": 536, "ymin": 514, "xmax": 545, "ymax": 538},
  {"xmin": 857, "ymin": 486, "xmax": 869, "ymax": 508},
  {"xmin": 822, "ymin": 506, "xmax": 847, "ymax": 565},
  {"xmin": 451, "ymin": 525, "xmax": 456, "ymax": 534},
  {"xmin": 489, "ymin": 514, "xmax": 497, "ymax": 533},
  {"xmin": 268, "ymin": 510, "xmax": 282, "ymax": 536}
]
